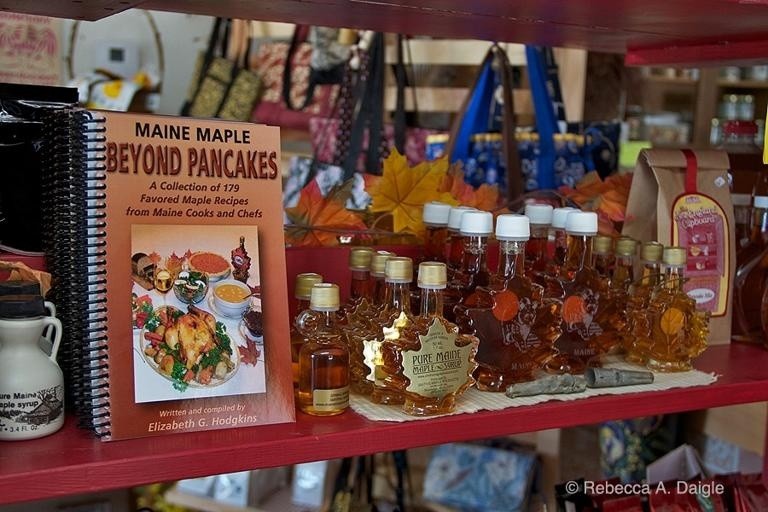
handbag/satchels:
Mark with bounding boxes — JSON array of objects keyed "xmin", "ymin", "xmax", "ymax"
[
  {"xmin": 421, "ymin": 436, "xmax": 538, "ymax": 511},
  {"xmin": 284, "ymin": 113, "xmax": 620, "ymax": 232},
  {"xmin": 177, "ymin": 45, "xmax": 266, "ymax": 127}
]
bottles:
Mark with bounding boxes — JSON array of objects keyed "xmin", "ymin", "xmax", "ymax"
[
  {"xmin": 293, "ymin": 191, "xmax": 768, "ymax": 419},
  {"xmin": 0, "ymin": 280, "xmax": 67, "ymax": 442}
]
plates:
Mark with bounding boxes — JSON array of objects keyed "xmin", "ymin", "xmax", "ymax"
[
  {"xmin": 142, "ymin": 318, "xmax": 240, "ymax": 385},
  {"xmin": 207, "ymin": 296, "xmax": 242, "ymax": 320},
  {"xmin": 172, "ymin": 270, "xmax": 208, "ymax": 305}
]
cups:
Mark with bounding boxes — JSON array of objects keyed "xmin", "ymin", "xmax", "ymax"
[
  {"xmin": 212, "ymin": 280, "xmax": 253, "ymax": 314},
  {"xmin": 625, "ymin": 61, "xmax": 768, "ymax": 146}
]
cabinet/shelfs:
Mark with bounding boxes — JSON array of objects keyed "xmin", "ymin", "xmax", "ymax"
[
  {"xmin": 0, "ymin": 1, "xmax": 767, "ymax": 507},
  {"xmin": 622, "ymin": 60, "xmax": 767, "ymax": 158}
]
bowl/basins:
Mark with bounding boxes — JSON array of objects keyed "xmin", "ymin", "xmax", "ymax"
[{"xmin": 187, "ymin": 250, "xmax": 230, "ymax": 282}]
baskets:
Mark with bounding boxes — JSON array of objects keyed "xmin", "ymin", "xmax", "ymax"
[{"xmin": 63, "ymin": 8, "xmax": 166, "ymax": 116}]
